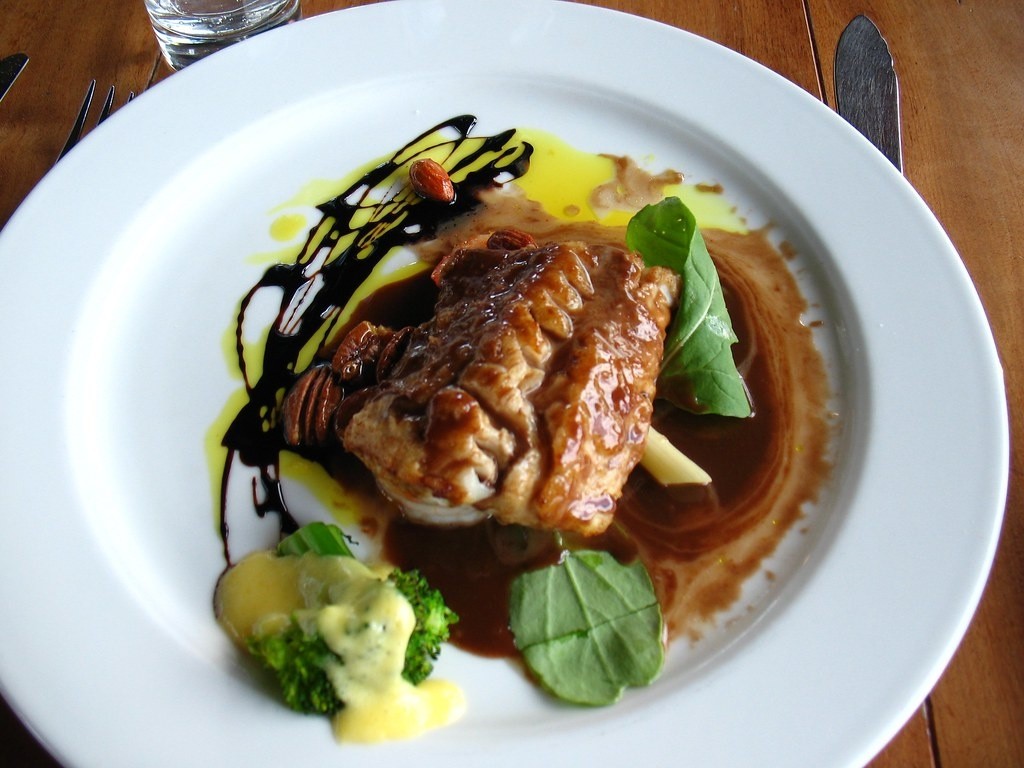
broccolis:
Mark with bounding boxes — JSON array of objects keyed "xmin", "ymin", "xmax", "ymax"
[{"xmin": 215, "ymin": 522, "xmax": 461, "ymax": 715}]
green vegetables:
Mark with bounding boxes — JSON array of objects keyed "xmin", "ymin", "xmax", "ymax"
[
  {"xmin": 505, "ymin": 532, "xmax": 666, "ymax": 706},
  {"xmin": 626, "ymin": 198, "xmax": 750, "ymax": 417}
]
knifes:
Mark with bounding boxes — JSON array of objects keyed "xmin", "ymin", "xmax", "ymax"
[
  {"xmin": 833, "ymin": 14, "xmax": 902, "ymax": 176},
  {"xmin": 0, "ymin": 52, "xmax": 29, "ymax": 102}
]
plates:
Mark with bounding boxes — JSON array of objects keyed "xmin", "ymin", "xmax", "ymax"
[{"xmin": 0, "ymin": 0, "xmax": 1010, "ymax": 768}]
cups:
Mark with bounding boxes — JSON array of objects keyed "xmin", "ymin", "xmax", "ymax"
[{"xmin": 143, "ymin": 0, "xmax": 303, "ymax": 73}]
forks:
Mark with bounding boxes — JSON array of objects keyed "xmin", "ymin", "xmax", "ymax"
[{"xmin": 53, "ymin": 80, "xmax": 136, "ymax": 166}]
